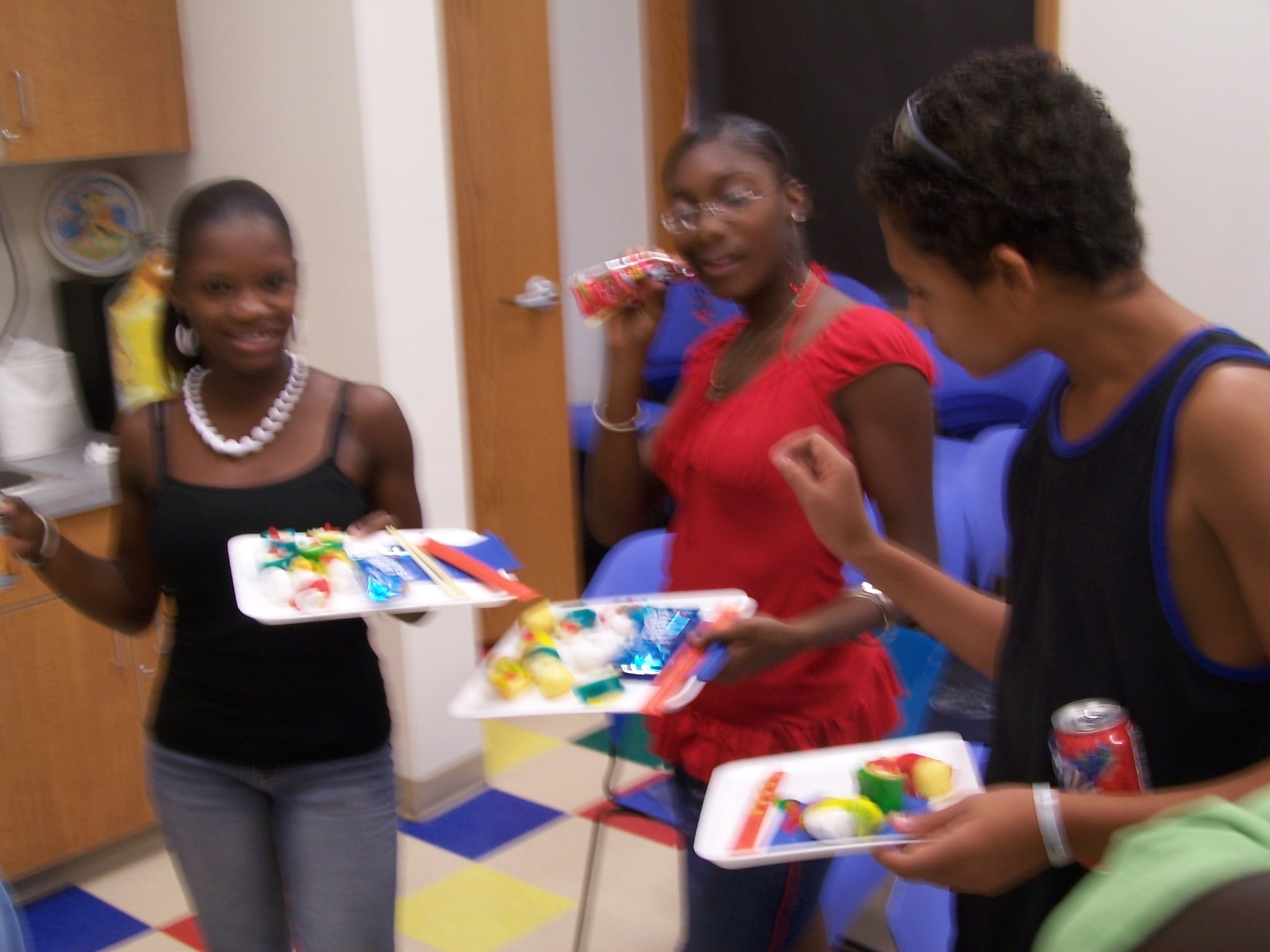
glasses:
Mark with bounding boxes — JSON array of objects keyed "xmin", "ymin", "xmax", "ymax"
[
  {"xmin": 893, "ymin": 88, "xmax": 1042, "ymax": 231},
  {"xmin": 660, "ymin": 187, "xmax": 785, "ymax": 235}
]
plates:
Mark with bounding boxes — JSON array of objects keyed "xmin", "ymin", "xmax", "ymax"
[
  {"xmin": 226, "ymin": 527, "xmax": 516, "ymax": 625},
  {"xmin": 694, "ymin": 732, "xmax": 989, "ymax": 870},
  {"xmin": 448, "ymin": 587, "xmax": 750, "ymax": 721}
]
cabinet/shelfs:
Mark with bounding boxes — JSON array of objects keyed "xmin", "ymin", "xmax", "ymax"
[
  {"xmin": 0, "ymin": 506, "xmax": 167, "ymax": 907},
  {"xmin": 0, "ymin": 0, "xmax": 191, "ymax": 169}
]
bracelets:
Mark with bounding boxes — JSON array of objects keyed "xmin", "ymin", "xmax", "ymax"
[
  {"xmin": 1031, "ymin": 781, "xmax": 1073, "ymax": 870},
  {"xmin": 841, "ymin": 582, "xmax": 896, "ymax": 647},
  {"xmin": 14, "ymin": 513, "xmax": 61, "ymax": 569},
  {"xmin": 590, "ymin": 400, "xmax": 650, "ymax": 433}
]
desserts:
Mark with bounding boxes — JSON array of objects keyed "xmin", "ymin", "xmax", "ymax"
[
  {"xmin": 489, "ymin": 600, "xmax": 626, "ymax": 707},
  {"xmin": 249, "ymin": 522, "xmax": 350, "ymax": 613},
  {"xmin": 800, "ymin": 753, "xmax": 954, "ymax": 840}
]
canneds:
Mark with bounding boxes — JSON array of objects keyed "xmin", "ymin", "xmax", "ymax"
[{"xmin": 1048, "ymin": 699, "xmax": 1151, "ymax": 871}]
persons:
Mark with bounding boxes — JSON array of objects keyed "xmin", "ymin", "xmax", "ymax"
[
  {"xmin": 0, "ymin": 177, "xmax": 430, "ymax": 952},
  {"xmin": 585, "ymin": 113, "xmax": 941, "ymax": 952},
  {"xmin": 857, "ymin": 48, "xmax": 1270, "ymax": 952}
]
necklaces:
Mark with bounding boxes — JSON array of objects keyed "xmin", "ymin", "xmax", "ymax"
[
  {"xmin": 182, "ymin": 350, "xmax": 309, "ymax": 455},
  {"xmin": 708, "ymin": 356, "xmax": 726, "ymax": 391}
]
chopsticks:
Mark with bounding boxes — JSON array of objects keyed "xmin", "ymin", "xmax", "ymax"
[{"xmin": 386, "ymin": 525, "xmax": 469, "ymax": 600}]
